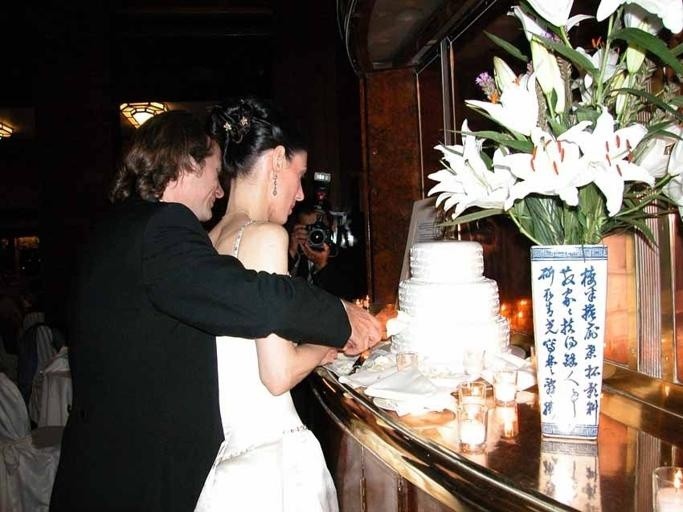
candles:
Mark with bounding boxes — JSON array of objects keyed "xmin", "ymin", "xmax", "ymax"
[
  {"xmin": 462, "ymin": 386, "xmax": 486, "ymax": 414},
  {"xmin": 495, "ymin": 371, "xmax": 517, "ymax": 402},
  {"xmin": 652, "ymin": 466, "xmax": 683, "ymax": 512}
]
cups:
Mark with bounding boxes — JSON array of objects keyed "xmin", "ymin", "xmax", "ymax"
[
  {"xmin": 458, "ymin": 371, "xmax": 520, "ymax": 457},
  {"xmin": 653, "ymin": 467, "xmax": 683, "ymax": 512},
  {"xmin": 397, "ymin": 352, "xmax": 417, "ymax": 372}
]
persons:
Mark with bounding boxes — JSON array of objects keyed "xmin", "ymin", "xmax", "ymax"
[
  {"xmin": 46, "ymin": 106, "xmax": 382, "ymax": 512},
  {"xmin": 191, "ymin": 100, "xmax": 340, "ymax": 512},
  {"xmin": 288, "ymin": 200, "xmax": 356, "ymax": 302}
]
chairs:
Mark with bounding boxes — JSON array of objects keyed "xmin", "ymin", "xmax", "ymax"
[{"xmin": 0, "ymin": 371, "xmax": 65, "ymax": 512}]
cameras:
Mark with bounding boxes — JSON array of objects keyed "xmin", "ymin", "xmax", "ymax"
[{"xmin": 306, "ymin": 212, "xmax": 332, "ymax": 250}]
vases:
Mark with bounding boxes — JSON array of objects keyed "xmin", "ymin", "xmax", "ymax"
[
  {"xmin": 530, "ymin": 246, "xmax": 608, "ymax": 439},
  {"xmin": 537, "ymin": 437, "xmax": 603, "ymax": 512}
]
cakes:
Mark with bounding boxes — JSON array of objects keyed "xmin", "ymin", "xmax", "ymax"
[{"xmin": 387, "ymin": 239, "xmax": 510, "ymax": 389}]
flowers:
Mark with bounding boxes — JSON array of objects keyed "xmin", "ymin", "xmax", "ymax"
[{"xmin": 423, "ymin": 0, "xmax": 683, "ymax": 247}]
